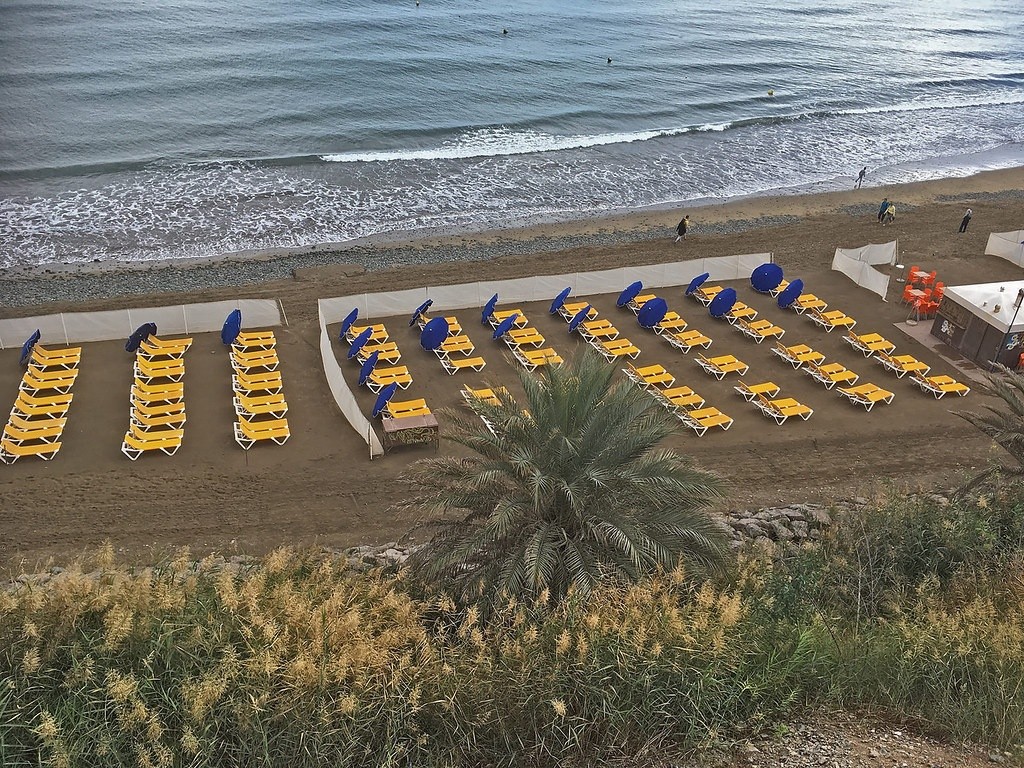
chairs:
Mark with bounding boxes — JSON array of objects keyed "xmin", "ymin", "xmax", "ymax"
[
  {"xmin": 232, "ymin": 375, "xmax": 283, "ymax": 397},
  {"xmin": 135, "ymin": 377, "xmax": 183, "ymax": 395},
  {"xmin": 0, "ymin": 343, "xmax": 82, "ymax": 465},
  {"xmin": 232, "ymin": 391, "xmax": 284, "ymax": 407},
  {"xmin": 230, "ymin": 354, "xmax": 280, "ymax": 374},
  {"xmin": 130, "ymin": 394, "xmax": 186, "ymax": 418},
  {"xmin": 233, "ymin": 414, "xmax": 289, "ymax": 432},
  {"xmin": 230, "ymin": 334, "xmax": 277, "ymax": 353},
  {"xmin": 229, "ymin": 346, "xmax": 278, "ymax": 361},
  {"xmin": 133, "ymin": 360, "xmax": 185, "ymax": 384},
  {"xmin": 239, "ymin": 330, "xmax": 275, "ymax": 340},
  {"xmin": 121, "ymin": 434, "xmax": 181, "ymax": 460},
  {"xmin": 231, "ymin": 367, "xmax": 281, "ymax": 383},
  {"xmin": 344, "ymin": 266, "xmax": 971, "ymax": 439},
  {"xmin": 125, "ymin": 424, "xmax": 185, "ymax": 442},
  {"xmin": 136, "ymin": 333, "xmax": 194, "ymax": 371},
  {"xmin": 235, "ymin": 396, "xmax": 288, "ymax": 422},
  {"xmin": 235, "ymin": 424, "xmax": 290, "ymax": 451},
  {"xmin": 130, "ymin": 407, "xmax": 186, "ymax": 432},
  {"xmin": 130, "ymin": 384, "xmax": 184, "ymax": 407}
]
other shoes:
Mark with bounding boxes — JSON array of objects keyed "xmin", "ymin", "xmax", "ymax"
[
  {"xmin": 883, "ymin": 224, "xmax": 885, "ymax": 226},
  {"xmin": 880, "ymin": 222, "xmax": 882, "ymax": 224}
]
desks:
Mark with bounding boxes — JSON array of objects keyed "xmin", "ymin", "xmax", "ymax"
[
  {"xmin": 381, "ymin": 414, "xmax": 440, "ymax": 454},
  {"xmin": 904, "ymin": 289, "xmax": 927, "ymax": 309},
  {"xmin": 911, "ymin": 271, "xmax": 930, "ymax": 289}
]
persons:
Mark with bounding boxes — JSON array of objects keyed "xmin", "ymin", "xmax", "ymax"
[
  {"xmin": 876, "ymin": 197, "xmax": 897, "ymax": 227},
  {"xmin": 855, "ymin": 166, "xmax": 867, "ymax": 187},
  {"xmin": 674, "ymin": 215, "xmax": 689, "ymax": 244},
  {"xmin": 958, "ymin": 208, "xmax": 972, "ymax": 233}
]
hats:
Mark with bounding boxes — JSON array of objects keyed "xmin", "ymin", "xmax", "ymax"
[{"xmin": 882, "ymin": 198, "xmax": 887, "ymax": 200}]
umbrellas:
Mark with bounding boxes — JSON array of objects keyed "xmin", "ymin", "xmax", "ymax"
[
  {"xmin": 548, "ymin": 262, "xmax": 806, "ymax": 335},
  {"xmin": 371, "ymin": 380, "xmax": 398, "ymax": 419},
  {"xmin": 358, "ymin": 349, "xmax": 379, "ymax": 387},
  {"xmin": 346, "ymin": 327, "xmax": 373, "ymax": 361},
  {"xmin": 125, "ymin": 322, "xmax": 157, "ymax": 353},
  {"xmin": 492, "ymin": 313, "xmax": 518, "ymax": 341},
  {"xmin": 339, "ymin": 307, "xmax": 359, "ymax": 339},
  {"xmin": 18, "ymin": 328, "xmax": 41, "ymax": 364},
  {"xmin": 408, "ymin": 298, "xmax": 433, "ymax": 327},
  {"xmin": 420, "ymin": 317, "xmax": 450, "ymax": 352},
  {"xmin": 481, "ymin": 292, "xmax": 499, "ymax": 326},
  {"xmin": 220, "ymin": 309, "xmax": 242, "ymax": 345}
]
